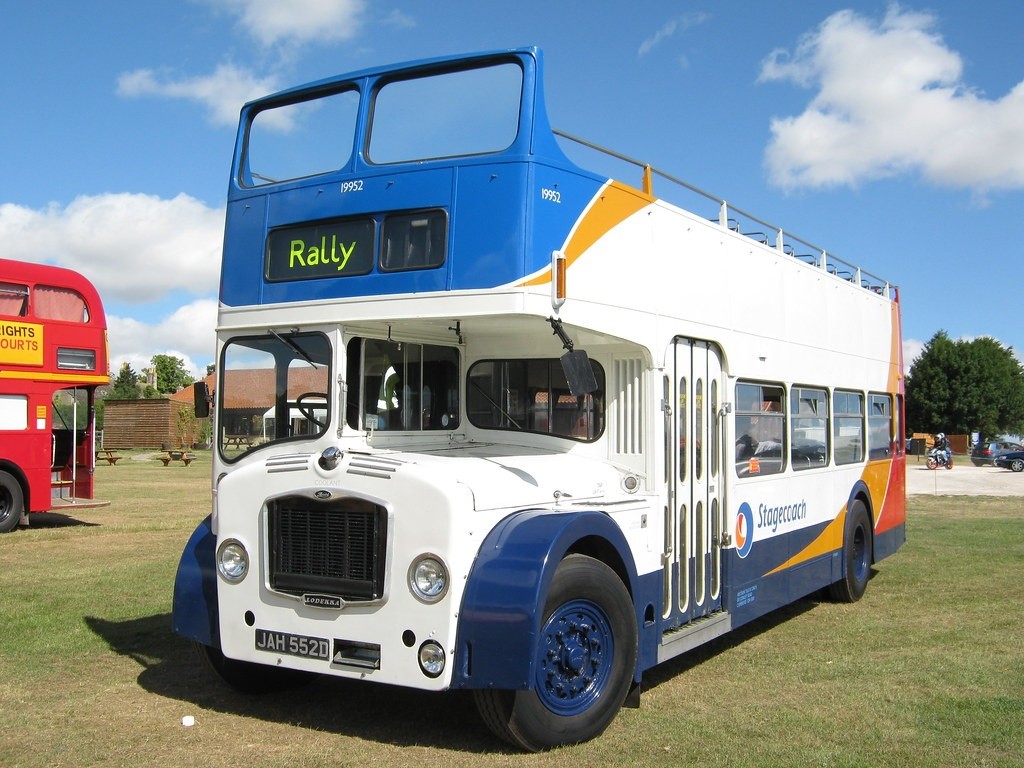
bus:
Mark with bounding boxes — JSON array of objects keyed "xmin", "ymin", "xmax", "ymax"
[
  {"xmin": 0, "ymin": 259, "xmax": 111, "ymax": 534},
  {"xmin": 170, "ymin": 48, "xmax": 907, "ymax": 755},
  {"xmin": 262, "ymin": 398, "xmax": 399, "ymax": 444}
]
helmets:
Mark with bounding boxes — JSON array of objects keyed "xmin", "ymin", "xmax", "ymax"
[
  {"xmin": 939, "ymin": 433, "xmax": 945, "ymax": 439},
  {"xmin": 934, "ymin": 434, "xmax": 941, "ymax": 442}
]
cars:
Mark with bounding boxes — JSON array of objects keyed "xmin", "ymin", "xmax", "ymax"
[
  {"xmin": 753, "ymin": 447, "xmax": 824, "ymax": 466},
  {"xmin": 905, "ymin": 437, "xmax": 911, "ymax": 451},
  {"xmin": 995, "ymin": 451, "xmax": 1024, "ymax": 472},
  {"xmin": 773, "ymin": 438, "xmax": 826, "ymax": 453},
  {"xmin": 970, "ymin": 442, "xmax": 1024, "ymax": 467},
  {"xmin": 734, "ymin": 456, "xmax": 801, "ymax": 478},
  {"xmin": 972, "ymin": 430, "xmax": 988, "ymax": 446}
]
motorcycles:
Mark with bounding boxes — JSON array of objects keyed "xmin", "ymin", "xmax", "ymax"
[{"xmin": 926, "ymin": 446, "xmax": 954, "ymax": 470}]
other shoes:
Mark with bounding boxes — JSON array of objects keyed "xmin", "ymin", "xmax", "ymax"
[{"xmin": 944, "ymin": 461, "xmax": 947, "ymax": 464}]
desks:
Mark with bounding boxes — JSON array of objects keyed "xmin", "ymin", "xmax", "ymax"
[
  {"xmin": 95, "ymin": 450, "xmax": 117, "ymax": 466},
  {"xmin": 224, "ymin": 435, "xmax": 251, "ymax": 452},
  {"xmin": 162, "ymin": 449, "xmax": 193, "ymax": 460}
]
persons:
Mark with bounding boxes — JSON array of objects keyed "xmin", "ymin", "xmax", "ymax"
[{"xmin": 934, "ymin": 432, "xmax": 952, "ymax": 465}]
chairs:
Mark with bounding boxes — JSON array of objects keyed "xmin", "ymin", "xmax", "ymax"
[
  {"xmin": 871, "ymin": 285, "xmax": 884, "ymax": 296},
  {"xmin": 854, "ymin": 280, "xmax": 871, "ymax": 291},
  {"xmin": 710, "ymin": 218, "xmax": 740, "ymax": 234},
  {"xmin": 738, "ymin": 430, "xmax": 888, "ymax": 477},
  {"xmin": 770, "ymin": 244, "xmax": 795, "ymax": 257},
  {"xmin": 794, "ymin": 254, "xmax": 818, "ymax": 266},
  {"xmin": 818, "ymin": 263, "xmax": 838, "ymax": 275},
  {"xmin": 837, "ymin": 271, "xmax": 854, "ymax": 283},
  {"xmin": 742, "ymin": 232, "xmax": 769, "ymax": 245}
]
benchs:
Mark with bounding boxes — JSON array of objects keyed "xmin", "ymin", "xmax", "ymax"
[
  {"xmin": 93, "ymin": 457, "xmax": 123, "ymax": 466},
  {"xmin": 157, "ymin": 458, "xmax": 172, "ymax": 467},
  {"xmin": 182, "ymin": 458, "xmax": 197, "ymax": 467},
  {"xmin": 225, "ymin": 442, "xmax": 254, "ymax": 445}
]
